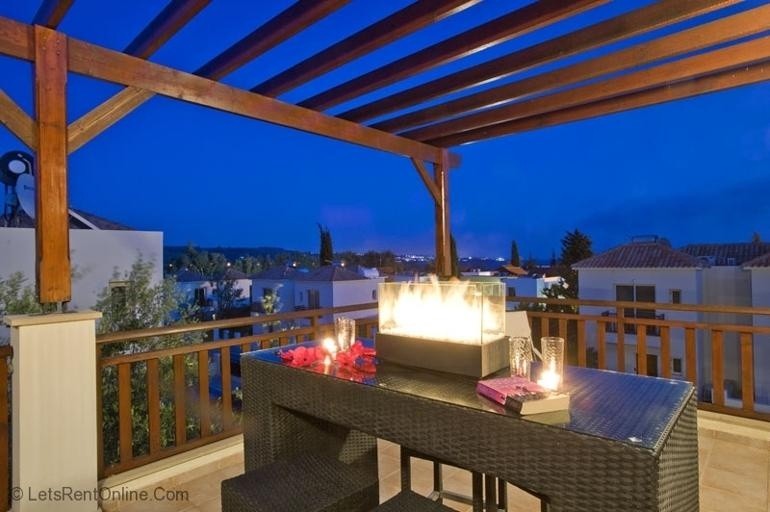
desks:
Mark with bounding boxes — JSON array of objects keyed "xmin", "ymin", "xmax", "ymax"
[{"xmin": 240, "ymin": 335, "xmax": 700, "ymax": 512}]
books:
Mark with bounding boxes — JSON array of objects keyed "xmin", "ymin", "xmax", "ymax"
[{"xmin": 475, "ymin": 375, "xmax": 571, "ymax": 419}]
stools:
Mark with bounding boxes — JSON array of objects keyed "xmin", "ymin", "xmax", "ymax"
[{"xmin": 222, "ymin": 448, "xmax": 460, "ymax": 512}]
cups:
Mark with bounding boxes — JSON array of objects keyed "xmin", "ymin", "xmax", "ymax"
[
  {"xmin": 541, "ymin": 337, "xmax": 565, "ymax": 390},
  {"xmin": 510, "ymin": 335, "xmax": 530, "ymax": 380},
  {"xmin": 337, "ymin": 318, "xmax": 356, "ymax": 357}
]
